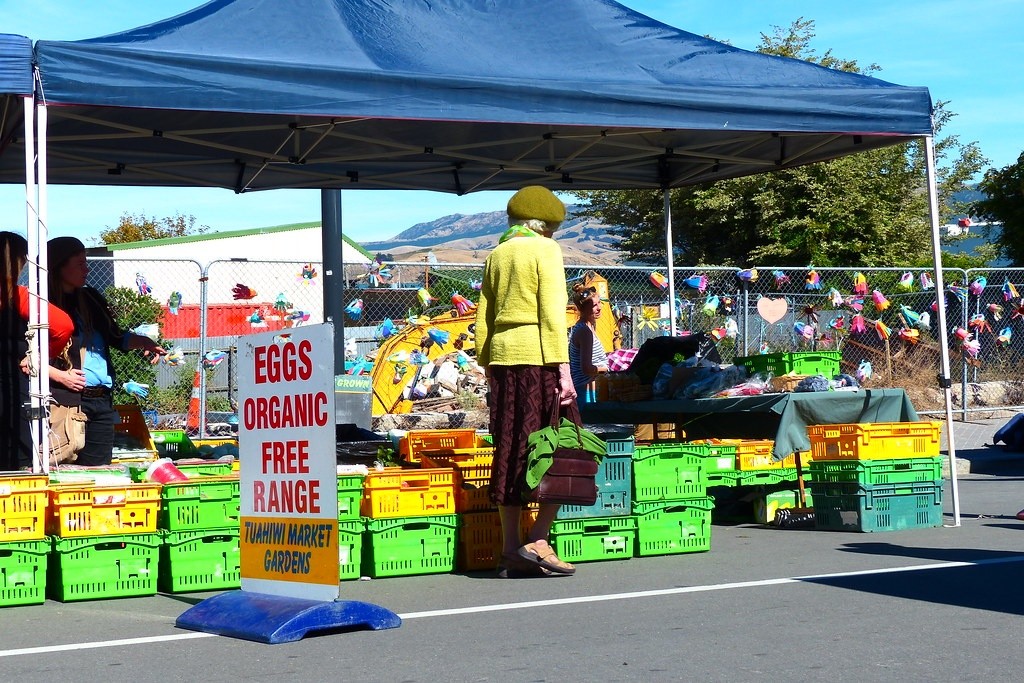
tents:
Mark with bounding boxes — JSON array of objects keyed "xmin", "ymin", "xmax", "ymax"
[{"xmin": 0, "ymin": 0, "xmax": 961, "ymax": 526}]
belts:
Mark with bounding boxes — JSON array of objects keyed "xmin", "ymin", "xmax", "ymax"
[{"xmin": 78, "ymin": 387, "xmax": 111, "ymax": 398}]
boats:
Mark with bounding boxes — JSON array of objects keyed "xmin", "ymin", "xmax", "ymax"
[{"xmin": 940, "ymin": 215, "xmax": 1005, "ymax": 236}]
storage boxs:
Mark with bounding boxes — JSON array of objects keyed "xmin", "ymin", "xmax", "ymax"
[
  {"xmin": 149, "ymin": 429, "xmax": 239, "ymax": 454},
  {"xmin": 549, "ymin": 419, "xmax": 946, "ymax": 564},
  {"xmin": 0, "ymin": 457, "xmax": 241, "ymax": 611},
  {"xmin": 336, "ymin": 428, "xmax": 497, "ymax": 581},
  {"xmin": 731, "ymin": 352, "xmax": 842, "ymax": 379}
]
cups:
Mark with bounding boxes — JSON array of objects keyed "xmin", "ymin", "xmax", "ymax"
[{"xmin": 144, "ymin": 458, "xmax": 190, "ymax": 484}]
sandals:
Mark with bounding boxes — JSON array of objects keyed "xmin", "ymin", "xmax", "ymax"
[
  {"xmin": 496, "ymin": 554, "xmax": 551, "ymax": 578},
  {"xmin": 516, "ymin": 536, "xmax": 576, "ymax": 573}
]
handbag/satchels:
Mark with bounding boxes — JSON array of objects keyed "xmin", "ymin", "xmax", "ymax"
[
  {"xmin": 528, "ymin": 391, "xmax": 599, "ymax": 506},
  {"xmin": 39, "ymin": 404, "xmax": 87, "ymax": 465}
]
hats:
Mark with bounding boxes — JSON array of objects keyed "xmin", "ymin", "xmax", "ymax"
[
  {"xmin": 572, "ymin": 284, "xmax": 597, "ymax": 310},
  {"xmin": 507, "ymin": 185, "xmax": 567, "ymax": 223}
]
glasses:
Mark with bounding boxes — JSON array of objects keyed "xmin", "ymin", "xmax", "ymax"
[{"xmin": 578, "ymin": 286, "xmax": 596, "ymax": 303}]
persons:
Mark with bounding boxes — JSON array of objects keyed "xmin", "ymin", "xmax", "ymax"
[
  {"xmin": 568, "ymin": 284, "xmax": 609, "ymax": 422},
  {"xmin": 1, "ymin": 230, "xmax": 76, "ymax": 471},
  {"xmin": 37, "ymin": 235, "xmax": 168, "ymax": 472},
  {"xmin": 473, "ymin": 184, "xmax": 577, "ymax": 580}
]
